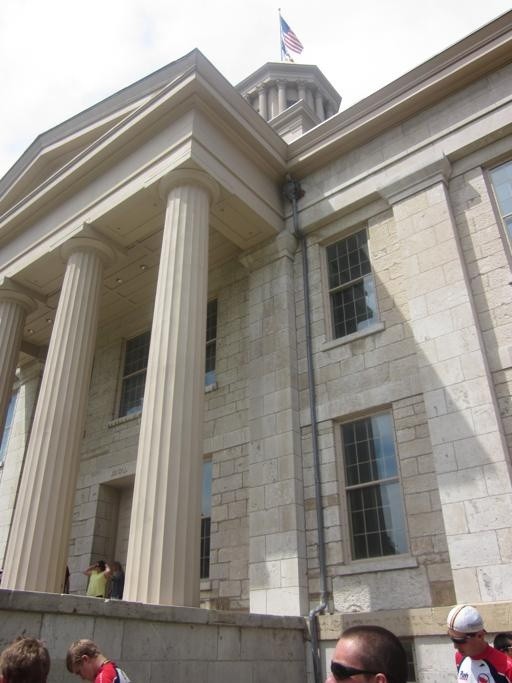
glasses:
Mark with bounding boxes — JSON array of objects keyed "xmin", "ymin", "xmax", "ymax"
[
  {"xmin": 450, "ymin": 636, "xmax": 472, "ymax": 644},
  {"xmin": 330, "ymin": 661, "xmax": 375, "ymax": 680}
]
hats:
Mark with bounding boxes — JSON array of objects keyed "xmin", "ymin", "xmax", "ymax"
[{"xmin": 446, "ymin": 602, "xmax": 483, "ymax": 634}]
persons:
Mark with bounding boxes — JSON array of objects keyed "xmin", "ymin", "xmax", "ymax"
[
  {"xmin": 64, "ymin": 566, "xmax": 70, "ymax": 594},
  {"xmin": 83, "ymin": 560, "xmax": 111, "ymax": 598},
  {"xmin": 494, "ymin": 632, "xmax": 512, "ymax": 657},
  {"xmin": 448, "ymin": 604, "xmax": 512, "ymax": 683},
  {"xmin": 108, "ymin": 561, "xmax": 125, "ymax": 599},
  {"xmin": 322, "ymin": 625, "xmax": 408, "ymax": 683},
  {"xmin": 0, "ymin": 635, "xmax": 51, "ymax": 683},
  {"xmin": 65, "ymin": 638, "xmax": 131, "ymax": 683}
]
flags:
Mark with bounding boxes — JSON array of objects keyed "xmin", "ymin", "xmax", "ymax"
[{"xmin": 280, "ymin": 16, "xmax": 304, "ymax": 54}]
